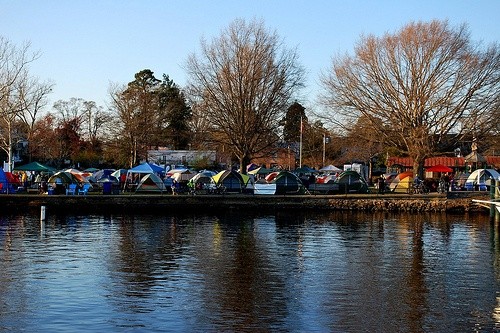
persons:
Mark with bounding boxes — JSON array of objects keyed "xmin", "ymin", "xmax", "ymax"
[{"xmin": 377, "ymin": 174, "xmax": 385, "ymax": 195}]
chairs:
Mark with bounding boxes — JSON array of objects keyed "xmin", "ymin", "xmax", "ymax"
[
  {"xmin": 479, "ymin": 183, "xmax": 487, "ymax": 191},
  {"xmin": 464, "ymin": 182, "xmax": 475, "ymax": 191},
  {"xmin": 65, "ymin": 183, "xmax": 77, "ymax": 196},
  {"xmin": 78, "ymin": 184, "xmax": 90, "ymax": 195}
]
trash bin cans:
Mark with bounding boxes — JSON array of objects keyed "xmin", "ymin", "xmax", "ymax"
[
  {"xmin": 113, "ymin": 184, "xmax": 119, "ymax": 195},
  {"xmin": 103, "ymin": 182, "xmax": 111, "ymax": 194}
]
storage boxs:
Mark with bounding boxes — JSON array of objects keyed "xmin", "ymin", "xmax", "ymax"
[{"xmin": 48, "ymin": 188, "xmax": 54, "ymax": 195}]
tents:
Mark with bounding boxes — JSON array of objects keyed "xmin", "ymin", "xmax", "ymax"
[{"xmin": 0, "ymin": 161, "xmax": 500, "ymax": 197}]
